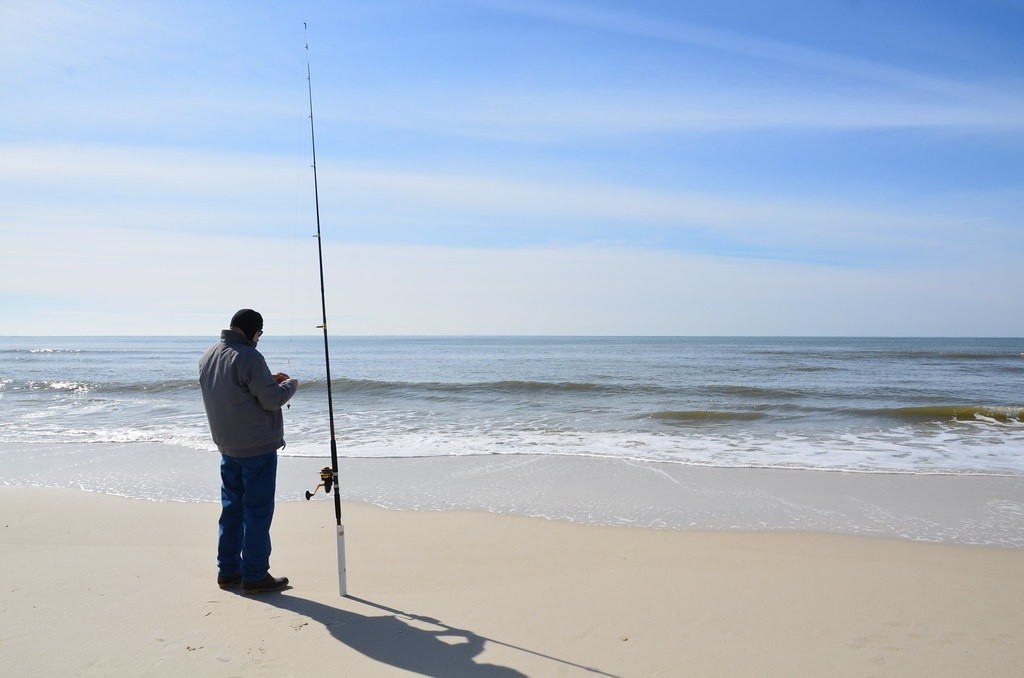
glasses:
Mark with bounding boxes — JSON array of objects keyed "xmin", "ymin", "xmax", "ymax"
[{"xmin": 258, "ymin": 329, "xmax": 263, "ymax": 337}]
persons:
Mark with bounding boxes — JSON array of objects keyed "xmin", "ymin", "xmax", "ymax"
[{"xmin": 198, "ymin": 309, "xmax": 299, "ymax": 596}]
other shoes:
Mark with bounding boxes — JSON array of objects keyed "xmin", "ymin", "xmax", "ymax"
[
  {"xmin": 217, "ymin": 574, "xmax": 243, "ymax": 590},
  {"xmin": 241, "ymin": 573, "xmax": 289, "ymax": 595}
]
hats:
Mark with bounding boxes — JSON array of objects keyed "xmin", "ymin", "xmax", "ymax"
[{"xmin": 229, "ymin": 308, "xmax": 264, "ymax": 341}]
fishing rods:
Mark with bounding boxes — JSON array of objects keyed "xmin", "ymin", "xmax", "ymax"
[{"xmin": 302, "ymin": 20, "xmax": 348, "ymax": 596}]
